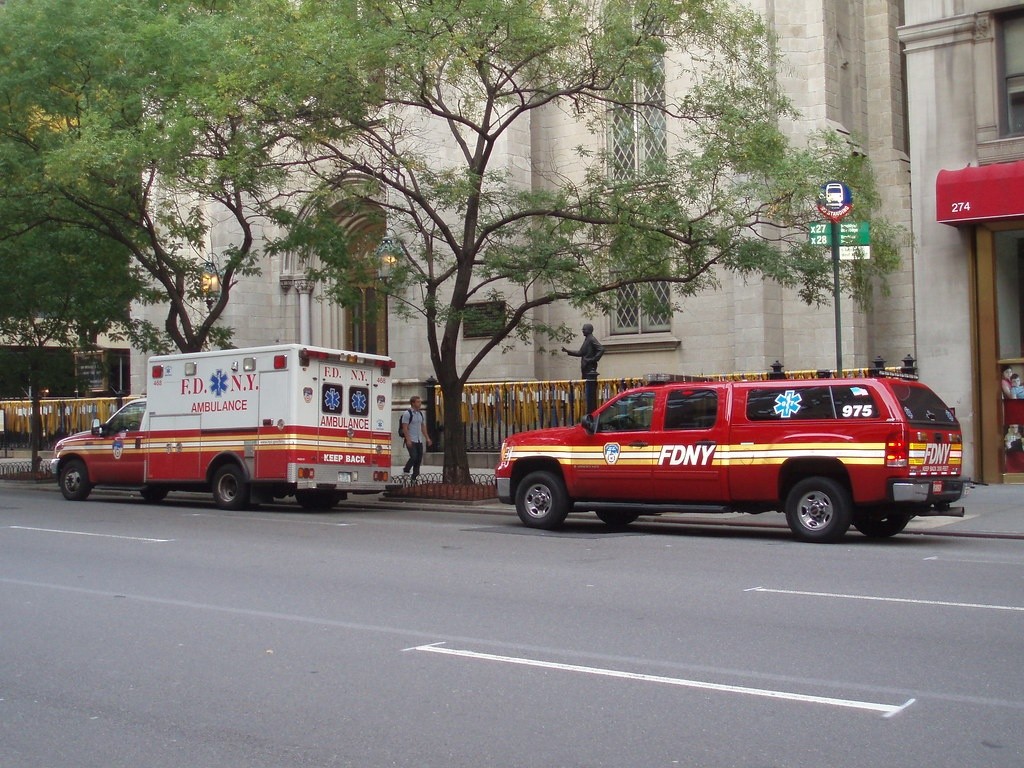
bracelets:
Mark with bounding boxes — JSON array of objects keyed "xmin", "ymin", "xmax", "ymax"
[{"xmin": 426, "ymin": 439, "xmax": 431, "ymax": 442}]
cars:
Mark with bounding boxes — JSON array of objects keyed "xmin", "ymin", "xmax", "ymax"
[{"xmin": 495, "ymin": 378, "xmax": 966, "ymax": 543}]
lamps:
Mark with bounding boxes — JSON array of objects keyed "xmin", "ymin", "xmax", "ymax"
[
  {"xmin": 378, "ymin": 228, "xmax": 406, "ymax": 288},
  {"xmin": 199, "ymin": 252, "xmax": 220, "ymax": 314}
]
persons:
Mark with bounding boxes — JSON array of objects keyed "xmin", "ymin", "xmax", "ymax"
[
  {"xmin": 1000, "ymin": 364, "xmax": 1024, "ymax": 400},
  {"xmin": 560, "ymin": 323, "xmax": 605, "ymax": 380},
  {"xmin": 1004, "ymin": 423, "xmax": 1024, "ymax": 452},
  {"xmin": 402, "ymin": 395, "xmax": 433, "ymax": 484}
]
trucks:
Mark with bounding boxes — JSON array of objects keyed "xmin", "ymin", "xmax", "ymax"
[{"xmin": 49, "ymin": 342, "xmax": 397, "ymax": 509}]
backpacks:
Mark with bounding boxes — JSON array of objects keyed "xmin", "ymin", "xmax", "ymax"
[{"xmin": 398, "ymin": 410, "xmax": 423, "ymax": 437}]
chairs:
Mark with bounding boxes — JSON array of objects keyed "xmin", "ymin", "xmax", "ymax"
[
  {"xmin": 706, "ymin": 406, "xmax": 717, "ymax": 427},
  {"xmin": 667, "ymin": 402, "xmax": 695, "ymax": 428}
]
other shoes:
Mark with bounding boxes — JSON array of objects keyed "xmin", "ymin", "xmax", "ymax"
[{"xmin": 401, "ymin": 468, "xmax": 409, "ymax": 476}]
